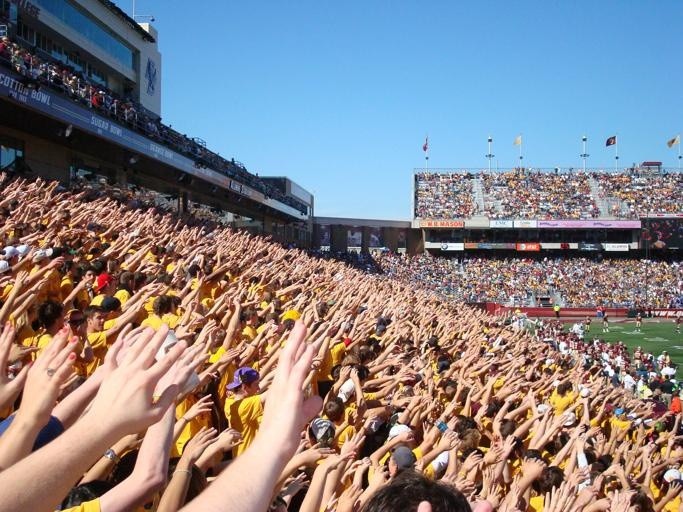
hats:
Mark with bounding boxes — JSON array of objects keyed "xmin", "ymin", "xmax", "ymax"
[
  {"xmin": 573, "ymin": 383, "xmax": 593, "ymax": 397},
  {"xmin": 306, "ymin": 417, "xmax": 335, "ymax": 446},
  {"xmin": 376, "ymin": 324, "xmax": 386, "ymax": 336},
  {"xmin": 92, "ymin": 271, "xmax": 114, "ymax": 292},
  {"xmin": 662, "ymin": 468, "xmax": 681, "ymax": 491},
  {"xmin": 0, "ymin": 259, "xmax": 11, "ymax": 274},
  {"xmin": 0, "ymin": 245, "xmax": 32, "ymax": 260},
  {"xmin": 438, "ymin": 360, "xmax": 450, "ymax": 369},
  {"xmin": 562, "ymin": 412, "xmax": 577, "ymax": 427},
  {"xmin": 31, "ymin": 248, "xmax": 54, "ymax": 264},
  {"xmin": 100, "ymin": 295, "xmax": 121, "ymax": 314},
  {"xmin": 388, "ymin": 422, "xmax": 416, "ymax": 475},
  {"xmin": 226, "ymin": 367, "xmax": 261, "ymax": 391},
  {"xmin": 336, "ymin": 378, "xmax": 355, "ymax": 403}
]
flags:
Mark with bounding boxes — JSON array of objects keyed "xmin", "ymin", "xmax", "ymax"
[
  {"xmin": 421, "ymin": 139, "xmax": 428, "ymax": 151},
  {"xmin": 513, "ymin": 135, "xmax": 520, "ymax": 145},
  {"xmin": 605, "ymin": 136, "xmax": 615, "ymax": 147},
  {"xmin": 666, "ymin": 135, "xmax": 679, "ymax": 148}
]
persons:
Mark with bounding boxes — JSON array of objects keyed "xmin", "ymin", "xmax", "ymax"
[
  {"xmin": 1, "ymin": 174, "xmax": 682, "ymax": 512},
  {"xmin": 0, "ymin": 32, "xmax": 247, "ymax": 173}
]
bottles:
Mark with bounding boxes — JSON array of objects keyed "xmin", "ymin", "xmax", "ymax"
[{"xmin": 434, "ymin": 419, "xmax": 448, "ymax": 433}]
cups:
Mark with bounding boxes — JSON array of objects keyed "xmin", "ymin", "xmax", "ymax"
[{"xmin": 152, "ymin": 330, "xmax": 200, "ymax": 393}]
